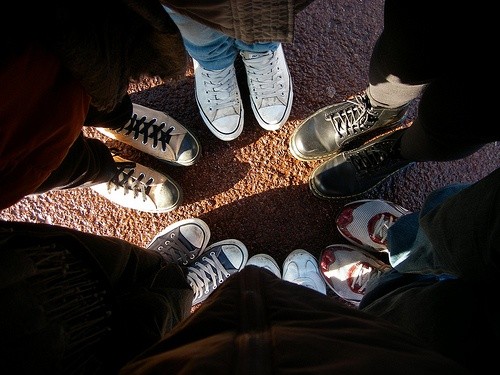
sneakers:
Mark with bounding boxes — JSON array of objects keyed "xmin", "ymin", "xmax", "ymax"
[
  {"xmin": 319, "ymin": 245, "xmax": 393, "ymax": 307},
  {"xmin": 245, "ymin": 253, "xmax": 281, "ymax": 280},
  {"xmin": 240, "ymin": 42, "xmax": 293, "ymax": 131},
  {"xmin": 89, "ymin": 153, "xmax": 183, "ymax": 213},
  {"xmin": 182, "ymin": 238, "xmax": 248, "ymax": 307},
  {"xmin": 93, "ymin": 103, "xmax": 202, "ymax": 166},
  {"xmin": 193, "ymin": 58, "xmax": 244, "ymax": 141},
  {"xmin": 282, "ymin": 249, "xmax": 327, "ymax": 295},
  {"xmin": 147, "ymin": 219, "xmax": 211, "ymax": 266},
  {"xmin": 335, "ymin": 199, "xmax": 413, "ymax": 252}
]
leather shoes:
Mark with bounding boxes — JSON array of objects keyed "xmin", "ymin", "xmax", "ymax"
[
  {"xmin": 309, "ymin": 127, "xmax": 411, "ymax": 201},
  {"xmin": 289, "ymin": 87, "xmax": 410, "ymax": 161}
]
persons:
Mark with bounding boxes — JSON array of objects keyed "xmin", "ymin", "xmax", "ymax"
[
  {"xmin": 122, "ymin": 249, "xmax": 468, "ymax": 375},
  {"xmin": 289, "ymin": 0, "xmax": 500, "ymax": 199},
  {"xmin": 318, "ymin": 167, "xmax": 500, "ymax": 375},
  {"xmin": 160, "ymin": 0, "xmax": 314, "ymax": 141},
  {"xmin": 0, "ymin": 217, "xmax": 249, "ymax": 375},
  {"xmin": 0, "ymin": 1, "xmax": 201, "ymax": 213}
]
